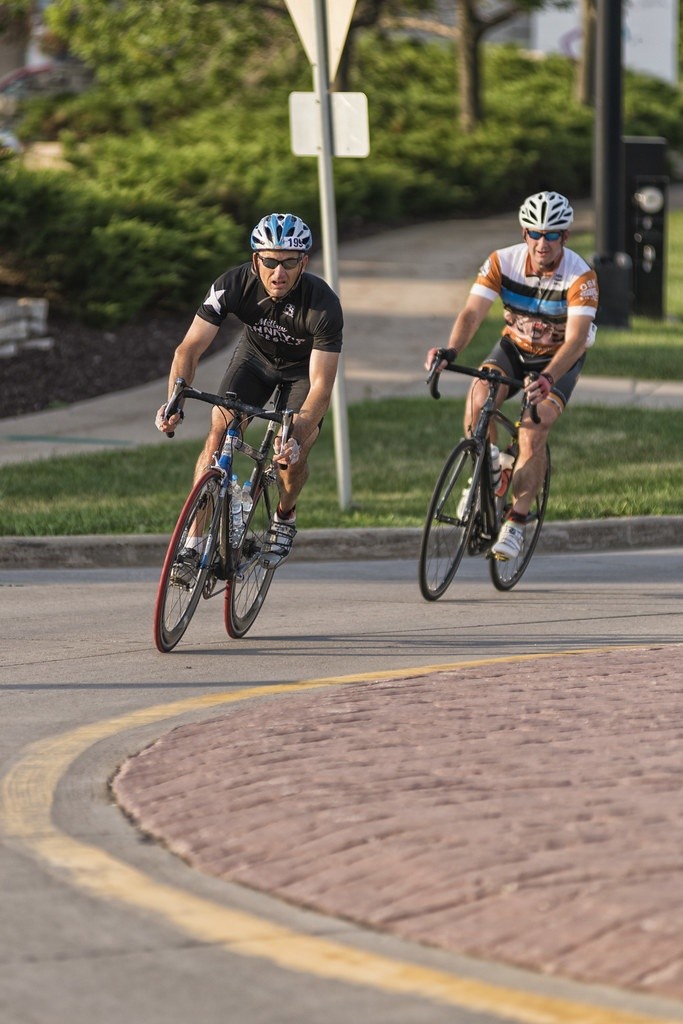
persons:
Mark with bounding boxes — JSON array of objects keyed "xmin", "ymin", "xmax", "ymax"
[
  {"xmin": 426, "ymin": 191, "xmax": 599, "ymax": 560},
  {"xmin": 154, "ymin": 213, "xmax": 344, "ymax": 589}
]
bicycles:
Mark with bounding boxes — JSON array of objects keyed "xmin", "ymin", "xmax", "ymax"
[
  {"xmin": 417, "ymin": 348, "xmax": 552, "ymax": 602},
  {"xmin": 153, "ymin": 374, "xmax": 294, "ymax": 653}
]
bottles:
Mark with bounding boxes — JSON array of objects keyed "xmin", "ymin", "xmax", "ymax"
[
  {"xmin": 240, "ymin": 479, "xmax": 253, "ymax": 524},
  {"xmin": 490, "ymin": 442, "xmax": 502, "ymax": 493},
  {"xmin": 231, "ymin": 474, "xmax": 242, "ymax": 526}
]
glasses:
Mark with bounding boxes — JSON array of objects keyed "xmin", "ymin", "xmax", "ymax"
[
  {"xmin": 525, "ymin": 228, "xmax": 563, "ymax": 241},
  {"xmin": 257, "ymin": 252, "xmax": 304, "ymax": 270}
]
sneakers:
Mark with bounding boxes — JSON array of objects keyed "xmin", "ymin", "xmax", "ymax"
[
  {"xmin": 456, "ymin": 478, "xmax": 478, "ymax": 522},
  {"xmin": 258, "ymin": 512, "xmax": 298, "ymax": 570},
  {"xmin": 170, "ymin": 547, "xmax": 201, "ymax": 590},
  {"xmin": 491, "ymin": 522, "xmax": 524, "ymax": 558}
]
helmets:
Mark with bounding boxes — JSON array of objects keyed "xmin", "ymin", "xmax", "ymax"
[
  {"xmin": 519, "ymin": 191, "xmax": 574, "ymax": 231},
  {"xmin": 251, "ymin": 213, "xmax": 312, "ymax": 251}
]
